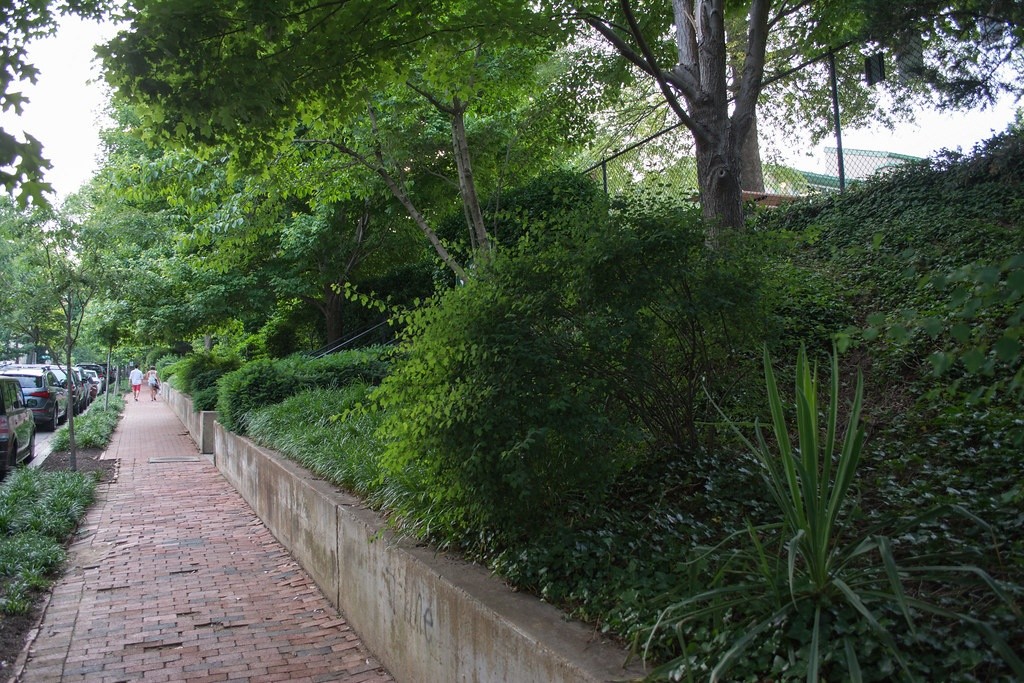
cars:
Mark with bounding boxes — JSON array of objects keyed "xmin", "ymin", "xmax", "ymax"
[
  {"xmin": 35, "ymin": 364, "xmax": 116, "ymax": 417},
  {"xmin": 0, "ymin": 370, "xmax": 68, "ymax": 431}
]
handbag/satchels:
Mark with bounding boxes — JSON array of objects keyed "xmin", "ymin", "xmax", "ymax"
[{"xmin": 155, "ymin": 383, "xmax": 161, "ymax": 390}]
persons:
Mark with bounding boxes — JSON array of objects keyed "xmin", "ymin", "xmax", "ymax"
[
  {"xmin": 145, "ymin": 365, "xmax": 160, "ymax": 402},
  {"xmin": 128, "ymin": 364, "xmax": 143, "ymax": 402}
]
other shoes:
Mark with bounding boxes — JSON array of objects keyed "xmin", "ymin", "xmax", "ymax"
[
  {"xmin": 134, "ymin": 396, "xmax": 138, "ymax": 401},
  {"xmin": 151, "ymin": 396, "xmax": 156, "ymax": 402}
]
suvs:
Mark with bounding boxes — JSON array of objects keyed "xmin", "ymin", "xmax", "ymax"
[{"xmin": 0, "ymin": 376, "xmax": 37, "ymax": 482}]
